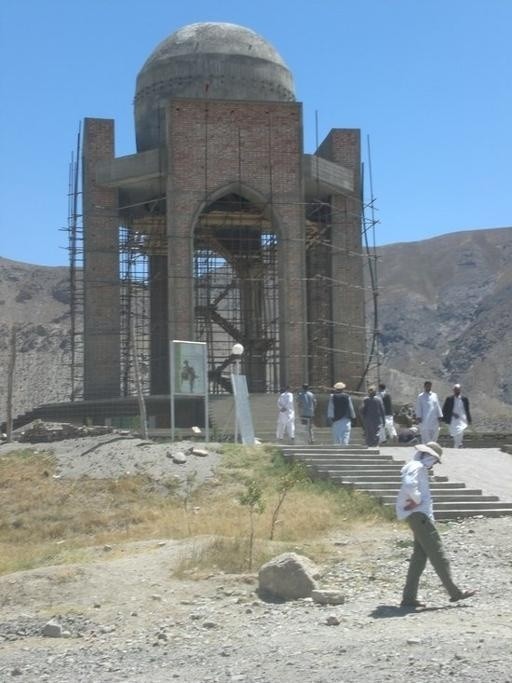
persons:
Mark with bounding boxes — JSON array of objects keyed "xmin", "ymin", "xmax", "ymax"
[
  {"xmin": 414, "ymin": 381, "xmax": 443, "ymax": 441},
  {"xmin": 394, "ymin": 441, "xmax": 476, "ymax": 607},
  {"xmin": 295, "ymin": 382, "xmax": 318, "ymax": 444},
  {"xmin": 373, "ymin": 383, "xmax": 398, "ymax": 443},
  {"xmin": 275, "ymin": 382, "xmax": 297, "ymax": 445},
  {"xmin": 326, "ymin": 382, "xmax": 356, "ymax": 446},
  {"xmin": 396, "ymin": 426, "xmax": 419, "ymax": 445},
  {"xmin": 360, "ymin": 385, "xmax": 386, "ymax": 445},
  {"xmin": 444, "ymin": 383, "xmax": 472, "ymax": 449}
]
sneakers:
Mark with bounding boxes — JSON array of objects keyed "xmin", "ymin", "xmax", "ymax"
[
  {"xmin": 398, "ymin": 598, "xmax": 428, "ymax": 609},
  {"xmin": 448, "ymin": 588, "xmax": 476, "ymax": 604}
]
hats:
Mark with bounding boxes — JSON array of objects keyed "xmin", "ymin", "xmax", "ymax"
[
  {"xmin": 414, "ymin": 440, "xmax": 445, "ymax": 464},
  {"xmin": 333, "ymin": 381, "xmax": 347, "ymax": 390}
]
